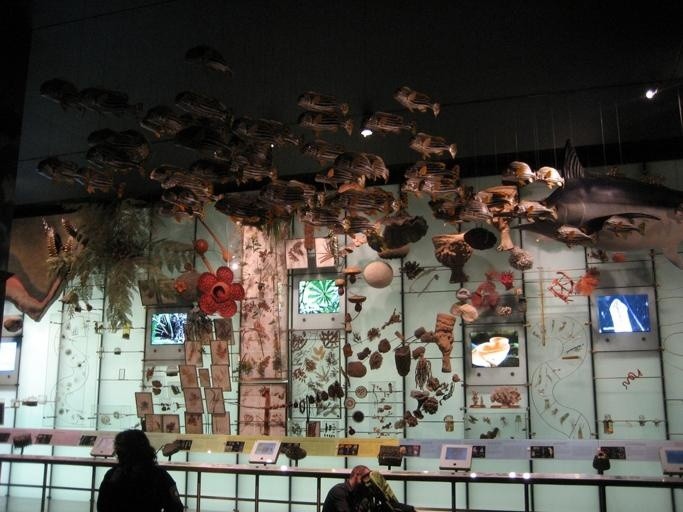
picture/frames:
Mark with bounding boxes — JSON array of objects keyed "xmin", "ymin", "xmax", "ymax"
[{"xmin": 135, "ymin": 317, "xmax": 235, "ymax": 435}]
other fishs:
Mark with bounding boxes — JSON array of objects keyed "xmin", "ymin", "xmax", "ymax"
[{"xmin": 34, "ymin": 83, "xmax": 682, "ymax": 272}]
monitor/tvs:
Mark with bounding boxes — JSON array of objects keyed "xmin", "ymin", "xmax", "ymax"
[
  {"xmin": 658, "ymin": 446, "xmax": 683, "ymax": 475},
  {"xmin": 249, "ymin": 440, "xmax": 282, "ymax": 464},
  {"xmin": 91, "ymin": 436, "xmax": 116, "ymax": 456},
  {"xmin": 439, "ymin": 444, "xmax": 473, "ymax": 470}
]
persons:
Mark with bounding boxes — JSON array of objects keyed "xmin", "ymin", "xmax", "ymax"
[
  {"xmin": 96, "ymin": 428, "xmax": 185, "ymax": 511},
  {"xmin": 321, "ymin": 464, "xmax": 376, "ymax": 511}
]
